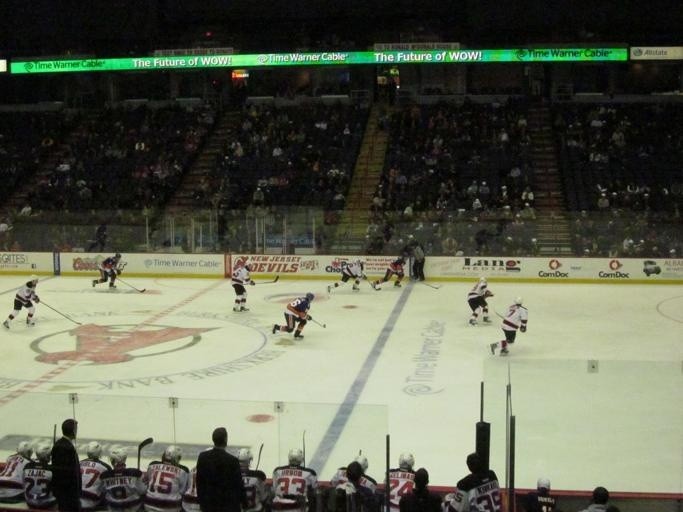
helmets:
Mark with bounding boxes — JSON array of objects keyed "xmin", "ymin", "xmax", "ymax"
[
  {"xmin": 307, "ymin": 293, "xmax": 314, "ymax": 300},
  {"xmin": 18, "ymin": 440, "xmax": 414, "ymax": 470},
  {"xmin": 480, "ymin": 278, "xmax": 487, "ymax": 289},
  {"xmin": 32, "ymin": 277, "xmax": 39, "ymax": 283}
]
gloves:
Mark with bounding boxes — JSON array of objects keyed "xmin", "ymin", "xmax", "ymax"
[
  {"xmin": 299, "ymin": 312, "xmax": 312, "ymax": 320},
  {"xmin": 250, "ymin": 281, "xmax": 255, "ymax": 285},
  {"xmin": 33, "ymin": 296, "xmax": 39, "ymax": 303},
  {"xmin": 484, "ymin": 290, "xmax": 491, "ymax": 297}
]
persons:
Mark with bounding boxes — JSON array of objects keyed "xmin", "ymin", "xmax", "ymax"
[
  {"xmin": 231, "ymin": 264, "xmax": 250, "ymax": 313},
  {"xmin": 489, "ymin": 296, "xmax": 529, "ymax": 354},
  {"xmin": 2, "ymin": 275, "xmax": 40, "ymax": 328},
  {"xmin": 272, "ymin": 292, "xmax": 314, "ymax": 339},
  {"xmin": 0, "ymin": 65, "xmax": 683, "ymax": 291},
  {"xmin": 0, "ymin": 416, "xmax": 620, "ymax": 512},
  {"xmin": 468, "ymin": 277, "xmax": 494, "ymax": 324}
]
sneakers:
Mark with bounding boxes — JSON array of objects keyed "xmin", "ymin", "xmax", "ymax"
[
  {"xmin": 501, "ymin": 348, "xmax": 508, "ymax": 354},
  {"xmin": 469, "ymin": 319, "xmax": 478, "ymax": 327},
  {"xmin": 239, "ymin": 307, "xmax": 249, "ymax": 312},
  {"xmin": 394, "ymin": 284, "xmax": 402, "ymax": 287},
  {"xmin": 233, "ymin": 307, "xmax": 241, "ymax": 312},
  {"xmin": 353, "ymin": 287, "xmax": 359, "ymax": 291},
  {"xmin": 374, "ymin": 281, "xmax": 379, "ymax": 289},
  {"xmin": 26, "ymin": 317, "xmax": 35, "ymax": 327},
  {"xmin": 109, "ymin": 283, "xmax": 116, "ymax": 288},
  {"xmin": 328, "ymin": 286, "xmax": 332, "ymax": 292},
  {"xmin": 294, "ymin": 330, "xmax": 303, "ymax": 339},
  {"xmin": 273, "ymin": 325, "xmax": 280, "ymax": 334},
  {"xmin": 483, "ymin": 317, "xmax": 492, "ymax": 323},
  {"xmin": 3, "ymin": 321, "xmax": 10, "ymax": 329},
  {"xmin": 490, "ymin": 344, "xmax": 498, "ymax": 355},
  {"xmin": 93, "ymin": 280, "xmax": 99, "ymax": 287}
]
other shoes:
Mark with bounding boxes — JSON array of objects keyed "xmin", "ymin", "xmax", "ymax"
[{"xmin": 414, "ymin": 277, "xmax": 425, "ymax": 281}]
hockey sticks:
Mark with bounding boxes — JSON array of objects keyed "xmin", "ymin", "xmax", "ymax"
[
  {"xmin": 311, "ymin": 316, "xmax": 327, "ymax": 328},
  {"xmin": 495, "ymin": 311, "xmax": 524, "ymax": 333},
  {"xmin": 302, "ymin": 429, "xmax": 307, "ymax": 466},
  {"xmin": 420, "ymin": 278, "xmax": 443, "ymax": 289},
  {"xmin": 366, "ymin": 277, "xmax": 381, "ymax": 292},
  {"xmin": 243, "ymin": 275, "xmax": 279, "ymax": 285},
  {"xmin": 116, "ymin": 276, "xmax": 145, "ymax": 292},
  {"xmin": 254, "ymin": 444, "xmax": 264, "ymax": 470},
  {"xmin": 38, "ymin": 299, "xmax": 84, "ymax": 325},
  {"xmin": 137, "ymin": 437, "xmax": 152, "ymax": 470}
]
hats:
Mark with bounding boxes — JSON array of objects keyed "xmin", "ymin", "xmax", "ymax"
[{"xmin": 537, "ymin": 477, "xmax": 550, "ymax": 489}]
